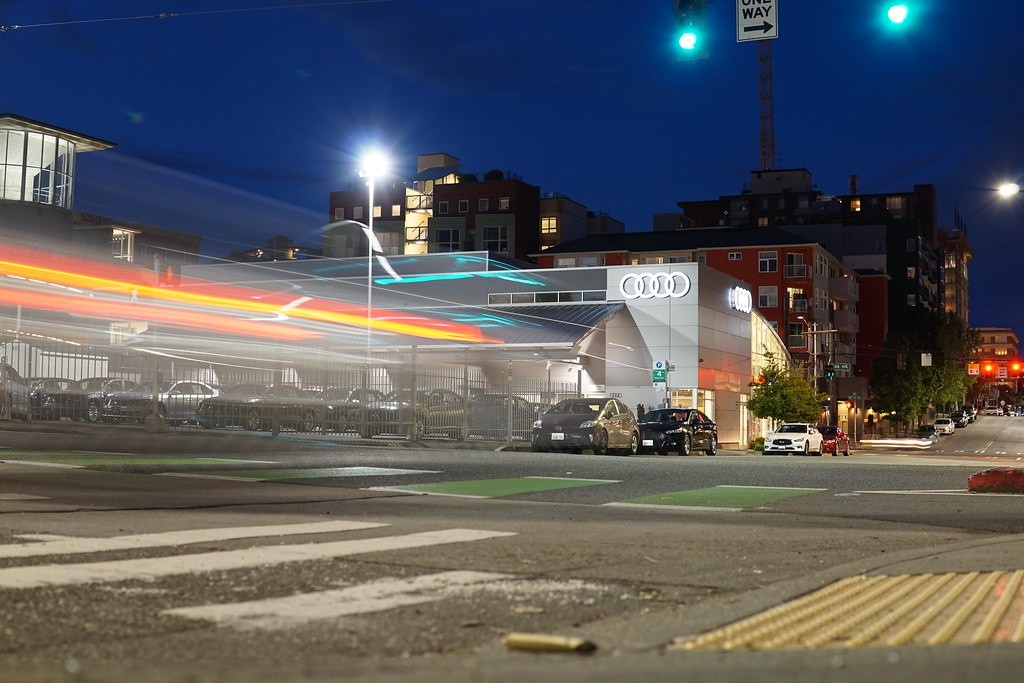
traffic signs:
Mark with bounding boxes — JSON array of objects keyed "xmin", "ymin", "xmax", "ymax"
[{"xmin": 736, "ymin": 0, "xmax": 779, "ymax": 44}]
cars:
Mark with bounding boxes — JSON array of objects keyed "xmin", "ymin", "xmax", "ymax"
[
  {"xmin": 960, "ymin": 404, "xmax": 978, "ymax": 423},
  {"xmin": 932, "ymin": 418, "xmax": 955, "ymax": 436},
  {"xmin": 530, "ymin": 396, "xmax": 642, "ymax": 458},
  {"xmin": 815, "ymin": 425, "xmax": 852, "ymax": 458},
  {"xmin": 763, "ymin": 423, "xmax": 824, "ymax": 457},
  {"xmin": 913, "ymin": 422, "xmax": 941, "ymax": 445},
  {"xmin": 637, "ymin": 407, "xmax": 718, "ymax": 458},
  {"xmin": 948, "ymin": 410, "xmax": 969, "ymax": 428},
  {"xmin": 1, "ymin": 357, "xmax": 555, "ymax": 447},
  {"xmin": 976, "ymin": 404, "xmax": 1022, "ymax": 418}
]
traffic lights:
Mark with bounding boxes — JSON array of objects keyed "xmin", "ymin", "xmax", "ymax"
[
  {"xmin": 676, "ymin": 0, "xmax": 710, "ymax": 63},
  {"xmin": 880, "ymin": 1, "xmax": 916, "ymax": 35}
]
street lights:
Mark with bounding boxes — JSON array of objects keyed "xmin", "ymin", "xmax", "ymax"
[
  {"xmin": 797, "ymin": 315, "xmax": 819, "ymax": 400},
  {"xmin": 360, "ymin": 144, "xmax": 391, "ymax": 374}
]
